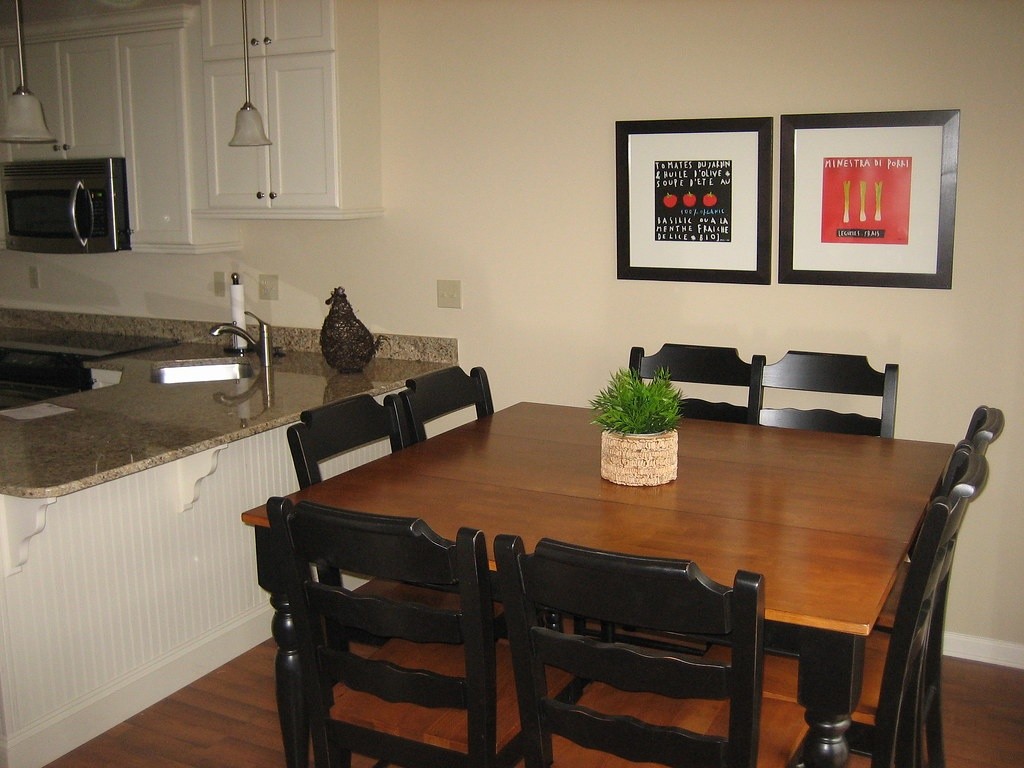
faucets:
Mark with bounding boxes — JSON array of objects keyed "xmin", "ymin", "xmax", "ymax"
[{"xmin": 209, "ymin": 310, "xmax": 273, "ymax": 367}]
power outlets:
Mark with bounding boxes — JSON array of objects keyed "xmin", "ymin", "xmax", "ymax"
[
  {"xmin": 258, "ymin": 274, "xmax": 280, "ymax": 301},
  {"xmin": 436, "ymin": 279, "xmax": 463, "ymax": 308},
  {"xmin": 214, "ymin": 270, "xmax": 226, "ymax": 297},
  {"xmin": 27, "ymin": 266, "xmax": 40, "ymax": 290}
]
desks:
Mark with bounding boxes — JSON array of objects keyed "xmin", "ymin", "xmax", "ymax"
[{"xmin": 241, "ymin": 400, "xmax": 956, "ymax": 768}]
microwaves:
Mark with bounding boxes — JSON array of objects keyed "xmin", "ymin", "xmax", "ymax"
[{"xmin": 0, "ymin": 156, "xmax": 130, "ymax": 254}]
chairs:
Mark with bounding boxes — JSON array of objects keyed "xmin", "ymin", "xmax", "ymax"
[
  {"xmin": 256, "ymin": 491, "xmax": 596, "ymax": 766},
  {"xmin": 492, "ymin": 534, "xmax": 782, "ymax": 764},
  {"xmin": 553, "ymin": 341, "xmax": 768, "ymax": 659},
  {"xmin": 870, "ymin": 405, "xmax": 1006, "ymax": 768},
  {"xmin": 701, "ymin": 447, "xmax": 992, "ymax": 768},
  {"xmin": 286, "ymin": 393, "xmax": 511, "ymax": 640},
  {"xmin": 392, "ymin": 364, "xmax": 567, "ymax": 633},
  {"xmin": 719, "ymin": 350, "xmax": 900, "ymax": 652}
]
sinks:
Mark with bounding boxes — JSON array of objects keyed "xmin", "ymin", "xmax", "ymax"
[{"xmin": 157, "ymin": 362, "xmax": 255, "ymax": 383}]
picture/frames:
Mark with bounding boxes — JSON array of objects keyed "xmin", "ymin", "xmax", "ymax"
[
  {"xmin": 775, "ymin": 105, "xmax": 964, "ymax": 289},
  {"xmin": 616, "ymin": 116, "xmax": 772, "ymax": 285}
]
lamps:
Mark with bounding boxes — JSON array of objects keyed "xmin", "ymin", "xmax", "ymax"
[
  {"xmin": 1, "ymin": 2, "xmax": 54, "ymax": 145},
  {"xmin": 225, "ymin": 2, "xmax": 273, "ymax": 147}
]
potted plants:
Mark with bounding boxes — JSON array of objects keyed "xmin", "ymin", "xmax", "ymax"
[{"xmin": 593, "ymin": 362, "xmax": 688, "ymax": 487}]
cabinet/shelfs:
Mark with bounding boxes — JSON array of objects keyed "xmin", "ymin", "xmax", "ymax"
[
  {"xmin": 8, "ymin": 33, "xmax": 125, "ymax": 159},
  {"xmin": 193, "ymin": 0, "xmax": 385, "ymax": 224},
  {"xmin": 118, "ymin": 27, "xmax": 244, "ymax": 253}
]
